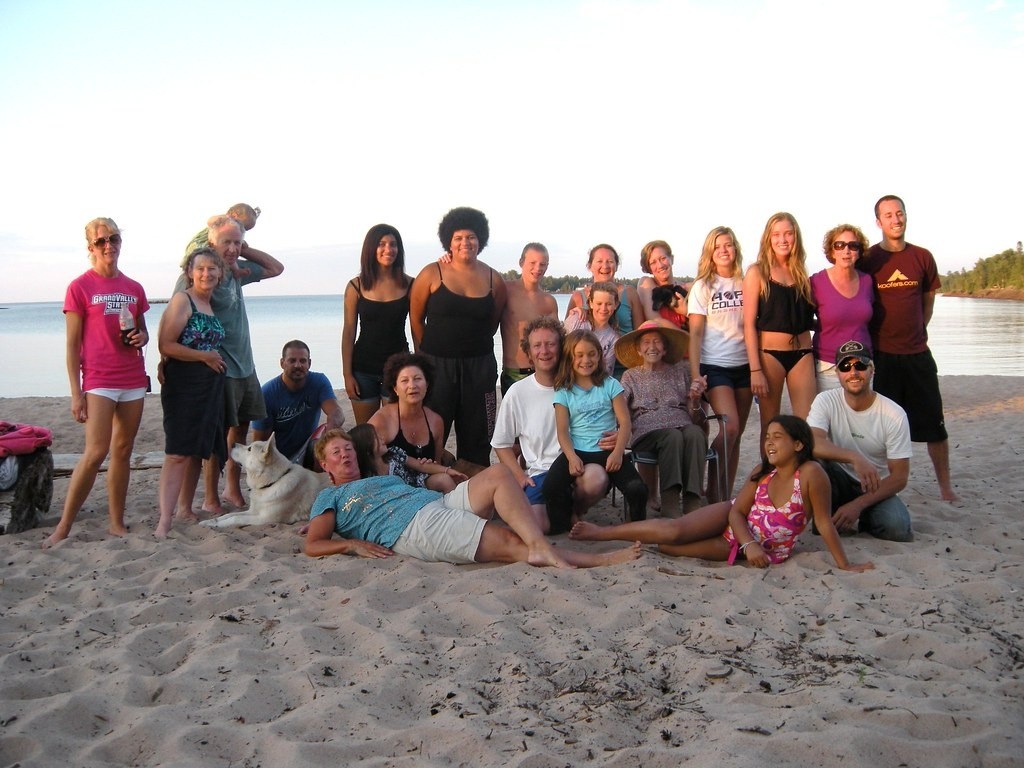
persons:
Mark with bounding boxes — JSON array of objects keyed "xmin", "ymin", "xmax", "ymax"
[
  {"xmin": 249, "ymin": 340, "xmax": 345, "ymax": 475},
  {"xmin": 42, "ymin": 217, "xmax": 151, "ymax": 550},
  {"xmin": 742, "ymin": 212, "xmax": 819, "ymax": 461},
  {"xmin": 341, "ymin": 223, "xmax": 454, "ymax": 425},
  {"xmin": 306, "ymin": 424, "xmax": 643, "ymax": 572},
  {"xmin": 439, "ymin": 226, "xmax": 760, "ymax": 533},
  {"xmin": 367, "ymin": 351, "xmax": 446, "ymax": 466},
  {"xmin": 409, "ymin": 206, "xmax": 508, "ymax": 464},
  {"xmin": 808, "ymin": 222, "xmax": 873, "ymax": 393},
  {"xmin": 806, "ymin": 337, "xmax": 913, "ymax": 542},
  {"xmin": 150, "ymin": 247, "xmax": 229, "ymax": 542},
  {"xmin": 157, "ymin": 203, "xmax": 284, "ymax": 516},
  {"xmin": 570, "ymin": 415, "xmax": 876, "ymax": 573},
  {"xmin": 855, "ymin": 195, "xmax": 972, "ymax": 502}
]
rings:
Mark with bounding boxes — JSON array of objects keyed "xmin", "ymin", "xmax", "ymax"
[{"xmin": 220, "ymin": 366, "xmax": 222, "ymax": 369}]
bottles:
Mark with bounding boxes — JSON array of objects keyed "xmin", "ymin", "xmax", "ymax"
[{"xmin": 118, "ymin": 303, "xmax": 137, "ymax": 347}]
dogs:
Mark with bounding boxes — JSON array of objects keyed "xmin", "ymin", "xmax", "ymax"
[{"xmin": 199, "ymin": 432, "xmax": 336, "ymax": 528}]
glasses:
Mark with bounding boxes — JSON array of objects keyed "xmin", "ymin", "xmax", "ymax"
[
  {"xmin": 837, "ymin": 360, "xmax": 869, "ymax": 371},
  {"xmin": 831, "ymin": 240, "xmax": 863, "ymax": 252},
  {"xmin": 90, "ymin": 233, "xmax": 122, "ymax": 248}
]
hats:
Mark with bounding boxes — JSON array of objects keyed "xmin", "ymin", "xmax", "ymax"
[
  {"xmin": 834, "ymin": 339, "xmax": 872, "ymax": 369},
  {"xmin": 612, "ymin": 319, "xmax": 691, "ymax": 368}
]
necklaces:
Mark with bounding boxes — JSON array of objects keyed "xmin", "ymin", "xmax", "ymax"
[{"xmin": 403, "ymin": 414, "xmax": 417, "ymax": 439}]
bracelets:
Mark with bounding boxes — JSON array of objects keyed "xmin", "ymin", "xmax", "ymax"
[
  {"xmin": 444, "ymin": 465, "xmax": 451, "ymax": 474},
  {"xmin": 692, "ymin": 404, "xmax": 702, "ymax": 410},
  {"xmin": 750, "ymin": 369, "xmax": 761, "ymax": 373},
  {"xmin": 739, "ymin": 540, "xmax": 758, "ymax": 553}
]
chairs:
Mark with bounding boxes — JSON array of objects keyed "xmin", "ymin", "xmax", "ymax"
[{"xmin": 611, "ymin": 412, "xmax": 730, "ymax": 523}]
